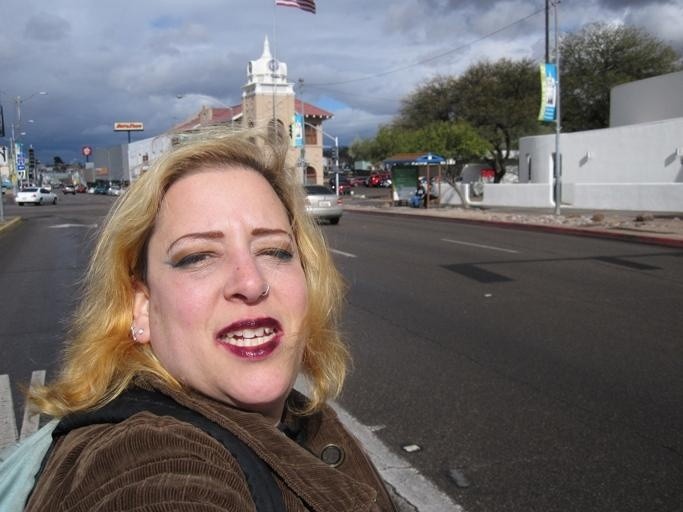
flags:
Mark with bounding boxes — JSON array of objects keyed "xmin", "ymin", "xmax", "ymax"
[{"xmin": 275, "ymin": 0, "xmax": 314, "ymax": 14}]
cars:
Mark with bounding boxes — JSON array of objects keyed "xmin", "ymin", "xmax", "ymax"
[
  {"xmin": 302, "ymin": 184, "xmax": 343, "ymax": 225},
  {"xmin": 62, "ymin": 185, "xmax": 124, "ymax": 195},
  {"xmin": 14, "ymin": 187, "xmax": 59, "ymax": 206},
  {"xmin": 330, "ymin": 170, "xmax": 392, "ymax": 196}
]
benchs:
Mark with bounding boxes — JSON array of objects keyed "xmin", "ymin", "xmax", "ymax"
[{"xmin": 394, "ymin": 197, "xmax": 439, "ymax": 207}]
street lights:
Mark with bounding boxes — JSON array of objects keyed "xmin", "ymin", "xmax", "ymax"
[
  {"xmin": 176, "ymin": 92, "xmax": 236, "ymax": 125},
  {"xmin": 9, "ymin": 90, "xmax": 48, "ymax": 197}
]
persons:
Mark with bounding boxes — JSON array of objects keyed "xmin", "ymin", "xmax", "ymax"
[
  {"xmin": 408, "ymin": 180, "xmax": 426, "ymax": 208},
  {"xmin": 421, "ymin": 176, "xmax": 439, "ymax": 207},
  {"xmin": 8, "ymin": 119, "xmax": 398, "ymax": 511}
]
